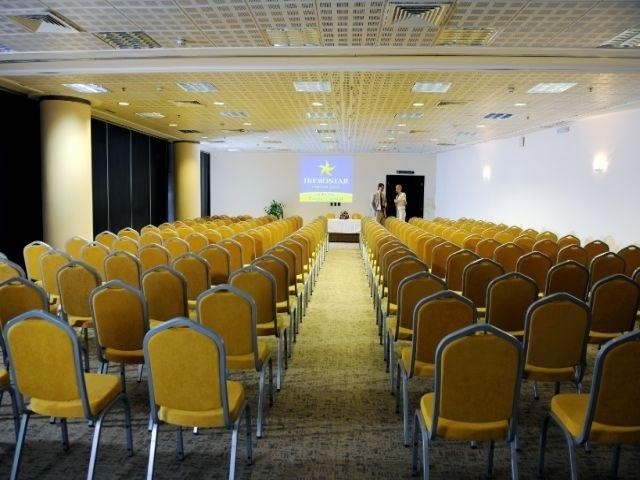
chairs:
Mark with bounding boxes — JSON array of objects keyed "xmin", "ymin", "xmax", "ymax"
[
  {"xmin": 143, "ymin": 317, "xmax": 252, "ymax": 479},
  {"xmin": 64, "ymin": 215, "xmax": 323, "ymax": 384},
  {"xmin": 2, "ymin": 241, "xmax": 75, "ymax": 454},
  {"xmin": 4, "ymin": 308, "xmax": 134, "ymax": 479},
  {"xmin": 618, "ymin": 244, "xmax": 640, "ymax": 271},
  {"xmin": 196, "ymin": 284, "xmax": 274, "ymax": 439},
  {"xmin": 546, "ymin": 259, "xmax": 591, "ymax": 302},
  {"xmin": 590, "ymin": 251, "xmax": 624, "ymax": 283},
  {"xmin": 479, "ymin": 272, "xmax": 539, "ymax": 339},
  {"xmin": 591, "ymin": 273, "xmax": 640, "ymax": 345},
  {"xmin": 395, "ymin": 290, "xmax": 478, "ymax": 449},
  {"xmin": 513, "ymin": 293, "xmax": 591, "ymax": 453},
  {"xmin": 361, "ymin": 217, "xmax": 608, "ymax": 360},
  {"xmin": 386, "ymin": 272, "xmax": 448, "ymax": 396},
  {"xmin": 88, "ymin": 279, "xmax": 149, "ymax": 392},
  {"xmin": 631, "ymin": 266, "xmax": 640, "ymax": 284},
  {"xmin": 411, "ymin": 323, "xmax": 524, "ymax": 479},
  {"xmin": 538, "ymin": 330, "xmax": 640, "ymax": 480}
]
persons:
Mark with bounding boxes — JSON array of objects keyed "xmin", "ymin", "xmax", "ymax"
[
  {"xmin": 370, "ymin": 183, "xmax": 387, "ymax": 223},
  {"xmin": 394, "ymin": 184, "xmax": 407, "ymax": 222}
]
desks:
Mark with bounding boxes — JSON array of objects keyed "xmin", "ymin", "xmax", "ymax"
[{"xmin": 324, "ymin": 217, "xmax": 362, "ymax": 242}]
